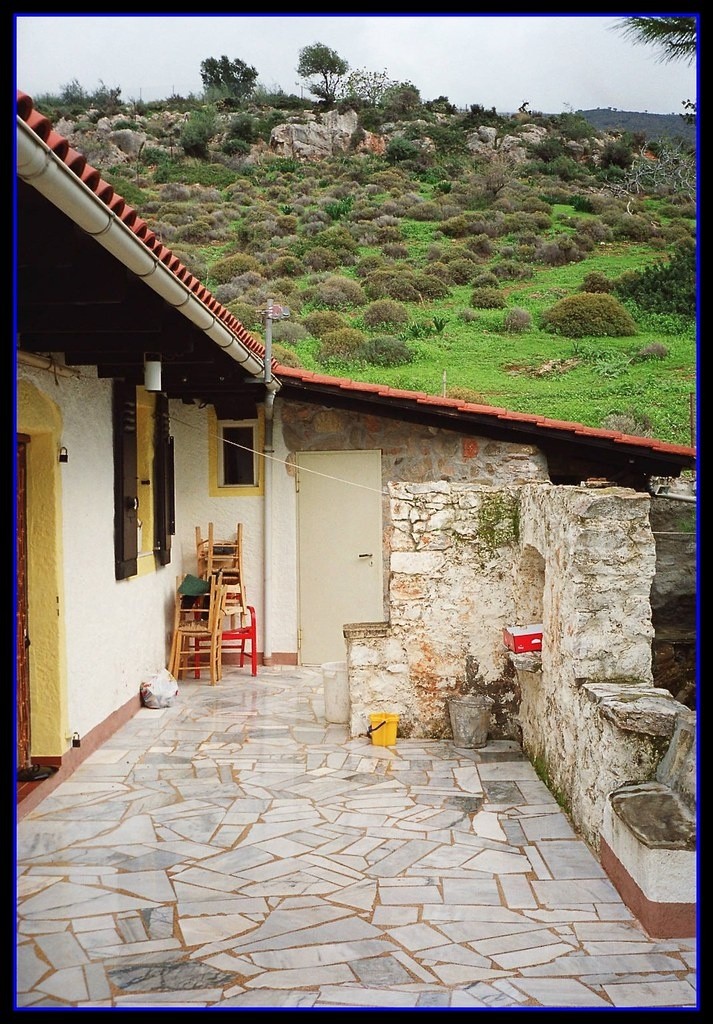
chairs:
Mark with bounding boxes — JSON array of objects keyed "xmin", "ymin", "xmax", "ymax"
[
  {"xmin": 200, "ymin": 538, "xmax": 246, "ymax": 629},
  {"xmin": 173, "ymin": 584, "xmax": 228, "ymax": 686},
  {"xmin": 194, "ymin": 586, "xmax": 257, "ymax": 679},
  {"xmin": 195, "ymin": 521, "xmax": 248, "ymax": 616},
  {"xmin": 169, "ymin": 573, "xmax": 218, "ymax": 672}
]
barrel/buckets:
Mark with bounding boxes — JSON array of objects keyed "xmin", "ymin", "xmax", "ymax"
[
  {"xmin": 321, "ymin": 661, "xmax": 350, "ymax": 724},
  {"xmin": 448, "ymin": 699, "xmax": 493, "ymax": 748},
  {"xmin": 368, "ymin": 712, "xmax": 398, "ymax": 745}
]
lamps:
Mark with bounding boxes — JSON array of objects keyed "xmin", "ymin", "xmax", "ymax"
[{"xmin": 143, "ymin": 351, "xmax": 163, "ymax": 392}]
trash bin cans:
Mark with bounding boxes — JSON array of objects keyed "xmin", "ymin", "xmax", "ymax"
[{"xmin": 320, "ymin": 660, "xmax": 350, "ymax": 724}]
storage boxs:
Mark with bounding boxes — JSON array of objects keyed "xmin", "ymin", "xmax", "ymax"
[{"xmin": 503, "ymin": 624, "xmax": 543, "ymax": 654}]
